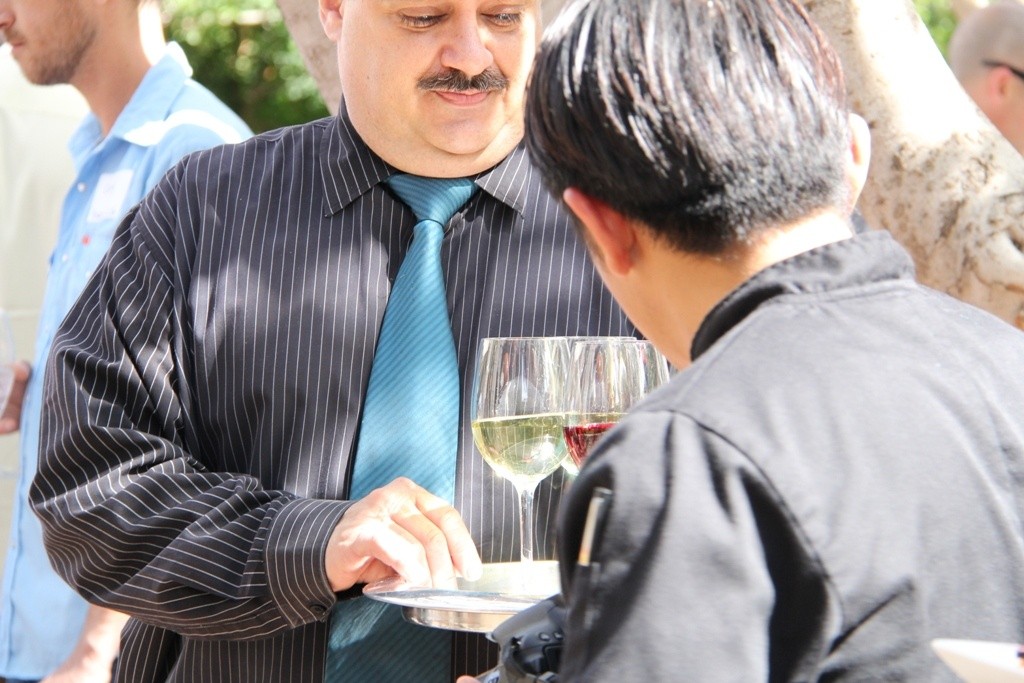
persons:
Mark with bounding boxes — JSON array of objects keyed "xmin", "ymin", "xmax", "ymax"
[
  {"xmin": 25, "ymin": 0, "xmax": 683, "ymax": 683},
  {"xmin": 946, "ymin": 0, "xmax": 1024, "ymax": 158},
  {"xmin": 453, "ymin": 0, "xmax": 1024, "ymax": 683},
  {"xmin": 0, "ymin": 0, "xmax": 256, "ymax": 683}
]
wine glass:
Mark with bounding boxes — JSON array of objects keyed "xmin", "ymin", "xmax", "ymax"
[{"xmin": 470, "ymin": 337, "xmax": 572, "ymax": 598}]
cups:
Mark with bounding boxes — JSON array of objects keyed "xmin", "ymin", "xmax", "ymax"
[
  {"xmin": 557, "ymin": 337, "xmax": 672, "ymax": 478},
  {"xmin": 0, "ymin": 308, "xmax": 16, "ymax": 419}
]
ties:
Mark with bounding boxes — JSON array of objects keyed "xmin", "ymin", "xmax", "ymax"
[{"xmin": 323, "ymin": 174, "xmax": 485, "ymax": 683}]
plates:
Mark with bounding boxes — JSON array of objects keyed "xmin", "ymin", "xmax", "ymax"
[{"xmin": 931, "ymin": 639, "xmax": 1024, "ymax": 683}]
